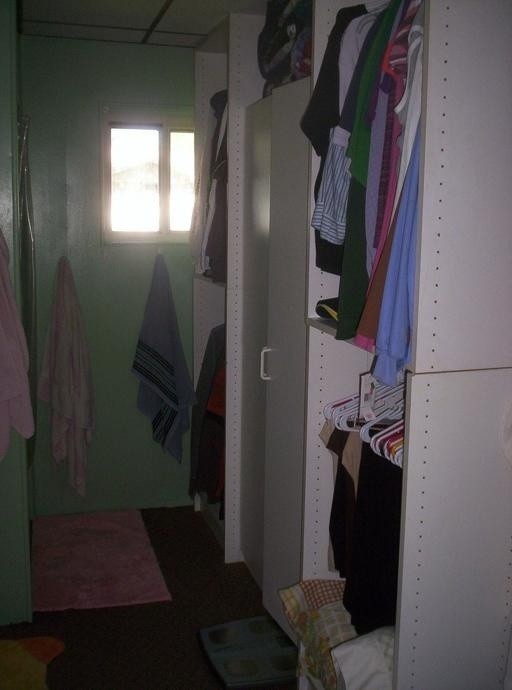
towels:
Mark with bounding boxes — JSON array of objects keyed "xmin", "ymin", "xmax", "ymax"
[
  {"xmin": 131, "ymin": 252, "xmax": 197, "ymax": 466},
  {"xmin": 35, "ymin": 255, "xmax": 95, "ymax": 507},
  {"xmin": 0, "ymin": 226, "xmax": 35, "ymax": 461}
]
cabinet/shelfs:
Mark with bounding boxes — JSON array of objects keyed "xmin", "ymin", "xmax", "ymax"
[
  {"xmin": 300, "ymin": 4, "xmax": 511, "ymax": 689},
  {"xmin": 192, "ymin": 13, "xmax": 264, "ymax": 564},
  {"xmin": 238, "ymin": 74, "xmax": 311, "ymax": 646}
]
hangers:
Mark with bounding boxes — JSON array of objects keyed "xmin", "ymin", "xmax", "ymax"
[{"xmin": 324, "ymin": 367, "xmax": 406, "ymax": 470}]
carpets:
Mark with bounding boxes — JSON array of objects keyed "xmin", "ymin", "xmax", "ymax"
[{"xmin": 30, "ymin": 510, "xmax": 171, "ymax": 611}]
